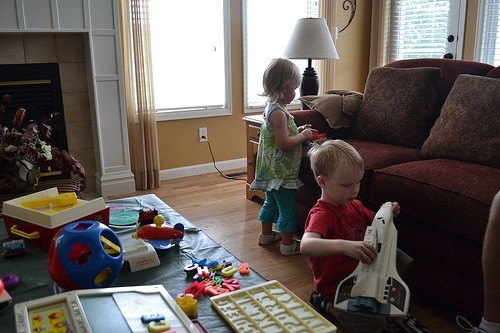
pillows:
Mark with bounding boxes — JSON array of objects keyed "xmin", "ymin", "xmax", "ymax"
[
  {"xmin": 297, "ymin": 94, "xmax": 354, "ymax": 129},
  {"xmin": 326, "ymin": 89, "xmax": 363, "ymax": 117},
  {"xmin": 358, "ymin": 67, "xmax": 443, "ymax": 149},
  {"xmin": 419, "ymin": 73, "xmax": 500, "ymax": 170}
]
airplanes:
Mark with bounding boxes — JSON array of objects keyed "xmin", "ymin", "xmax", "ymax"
[
  {"xmin": 131, "ymin": 214, "xmax": 186, "ymax": 251},
  {"xmin": 334, "ymin": 200, "xmax": 412, "ymax": 319}
]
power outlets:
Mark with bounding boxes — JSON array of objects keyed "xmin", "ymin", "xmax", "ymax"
[{"xmin": 199, "ymin": 127, "xmax": 207, "ymax": 142}]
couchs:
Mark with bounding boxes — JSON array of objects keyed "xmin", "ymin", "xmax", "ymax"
[{"xmin": 288, "ymin": 56, "xmax": 500, "ymax": 333}]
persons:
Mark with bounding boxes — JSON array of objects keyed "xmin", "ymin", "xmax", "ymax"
[
  {"xmin": 468, "ymin": 191, "xmax": 500, "ymax": 333},
  {"xmin": 249, "ymin": 59, "xmax": 318, "ymax": 256},
  {"xmin": 300, "ymin": 139, "xmax": 401, "ymax": 333}
]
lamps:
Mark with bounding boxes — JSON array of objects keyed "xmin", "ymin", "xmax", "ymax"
[{"xmin": 282, "ymin": 17, "xmax": 340, "ymax": 110}]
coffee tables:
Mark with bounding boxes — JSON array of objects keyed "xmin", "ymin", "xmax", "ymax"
[{"xmin": 0, "ymin": 194, "xmax": 269, "ymax": 333}]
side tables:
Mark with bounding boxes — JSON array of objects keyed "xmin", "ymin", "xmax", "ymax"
[{"xmin": 242, "ymin": 115, "xmax": 266, "ymax": 201}]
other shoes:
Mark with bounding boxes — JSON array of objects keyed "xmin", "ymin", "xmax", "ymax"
[
  {"xmin": 257, "ymin": 230, "xmax": 281, "ymax": 246},
  {"xmin": 279, "ymin": 238, "xmax": 302, "ymax": 255},
  {"xmin": 309, "ymin": 291, "xmax": 331, "ymax": 312}
]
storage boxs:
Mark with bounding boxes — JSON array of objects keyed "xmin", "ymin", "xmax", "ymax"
[{"xmin": 1, "ymin": 186, "xmax": 110, "ymax": 256}]
